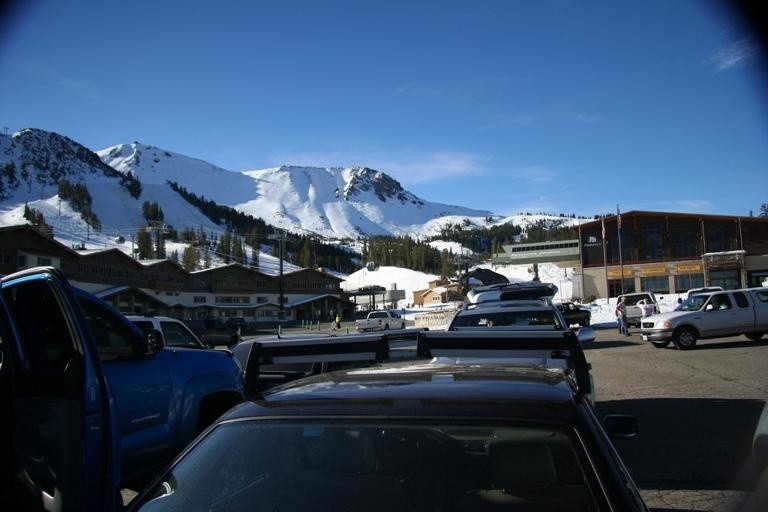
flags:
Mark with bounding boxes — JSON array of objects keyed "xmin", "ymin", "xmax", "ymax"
[
  {"xmin": 617, "ymin": 207, "xmax": 622, "ymax": 229},
  {"xmin": 602, "ymin": 221, "xmax": 605, "ymax": 240}
]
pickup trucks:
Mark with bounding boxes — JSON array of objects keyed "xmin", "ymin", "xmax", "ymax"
[
  {"xmin": 356, "ymin": 311, "xmax": 406, "ymax": 331},
  {"xmin": 636, "ymin": 288, "xmax": 766, "ymax": 350},
  {"xmin": 415, "ymin": 281, "xmax": 595, "ymax": 400}
]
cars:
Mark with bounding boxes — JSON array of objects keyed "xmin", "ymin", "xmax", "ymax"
[
  {"xmin": 136, "ymin": 361, "xmax": 651, "ymax": 512},
  {"xmin": 226, "ymin": 318, "xmax": 247, "ymax": 333},
  {"xmin": 182, "ymin": 318, "xmax": 242, "ymax": 351},
  {"xmin": 123, "ymin": 314, "xmax": 207, "ymax": 349}
]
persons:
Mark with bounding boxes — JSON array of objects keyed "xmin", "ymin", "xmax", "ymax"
[
  {"xmin": 616, "ymin": 297, "xmax": 632, "ymax": 336},
  {"xmin": 636, "ymin": 298, "xmax": 655, "ymax": 319},
  {"xmin": 335, "ymin": 314, "xmax": 342, "ymax": 328}
]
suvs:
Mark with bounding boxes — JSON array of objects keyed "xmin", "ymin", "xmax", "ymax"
[
  {"xmin": 685, "ymin": 287, "xmax": 725, "ymax": 303},
  {"xmin": 615, "ymin": 292, "xmax": 661, "ymax": 329},
  {"xmin": 1, "ymin": 264, "xmax": 245, "ymax": 512}
]
